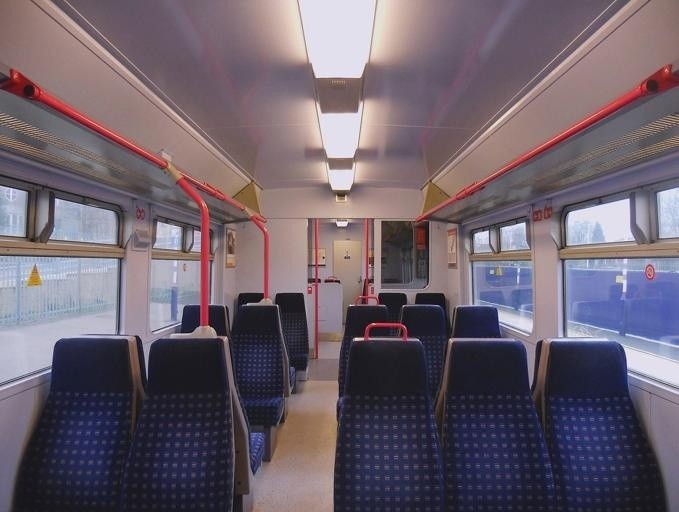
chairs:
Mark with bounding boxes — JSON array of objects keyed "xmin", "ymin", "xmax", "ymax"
[
  {"xmin": 10, "ymin": 288, "xmax": 311, "ymax": 512},
  {"xmin": 332, "ymin": 291, "xmax": 668, "ymax": 512}
]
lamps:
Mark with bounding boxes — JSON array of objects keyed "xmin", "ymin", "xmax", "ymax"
[{"xmin": 296, "ymin": 1, "xmax": 378, "ymax": 194}]
